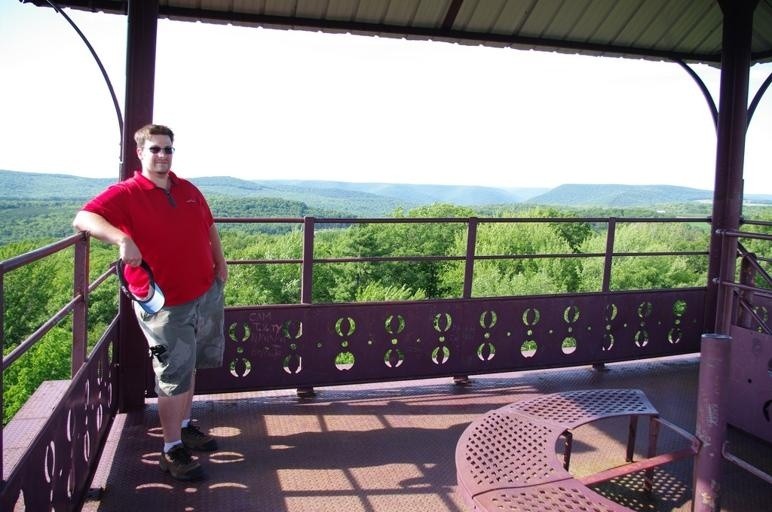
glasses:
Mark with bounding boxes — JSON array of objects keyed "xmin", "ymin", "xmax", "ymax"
[{"xmin": 135, "ymin": 145, "xmax": 174, "ymax": 154}]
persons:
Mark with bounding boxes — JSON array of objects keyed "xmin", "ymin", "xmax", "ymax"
[{"xmin": 73, "ymin": 123, "xmax": 228, "ymax": 480}]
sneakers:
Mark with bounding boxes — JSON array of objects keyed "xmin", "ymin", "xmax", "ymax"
[
  {"xmin": 179, "ymin": 421, "xmax": 218, "ymax": 452},
  {"xmin": 157, "ymin": 441, "xmax": 207, "ymax": 483}
]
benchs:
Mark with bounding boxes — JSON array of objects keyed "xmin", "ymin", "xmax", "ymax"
[{"xmin": 449, "ymin": 384, "xmax": 771, "ymax": 511}]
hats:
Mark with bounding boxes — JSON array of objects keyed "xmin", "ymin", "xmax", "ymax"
[{"xmin": 114, "ymin": 256, "xmax": 168, "ymax": 314}]
